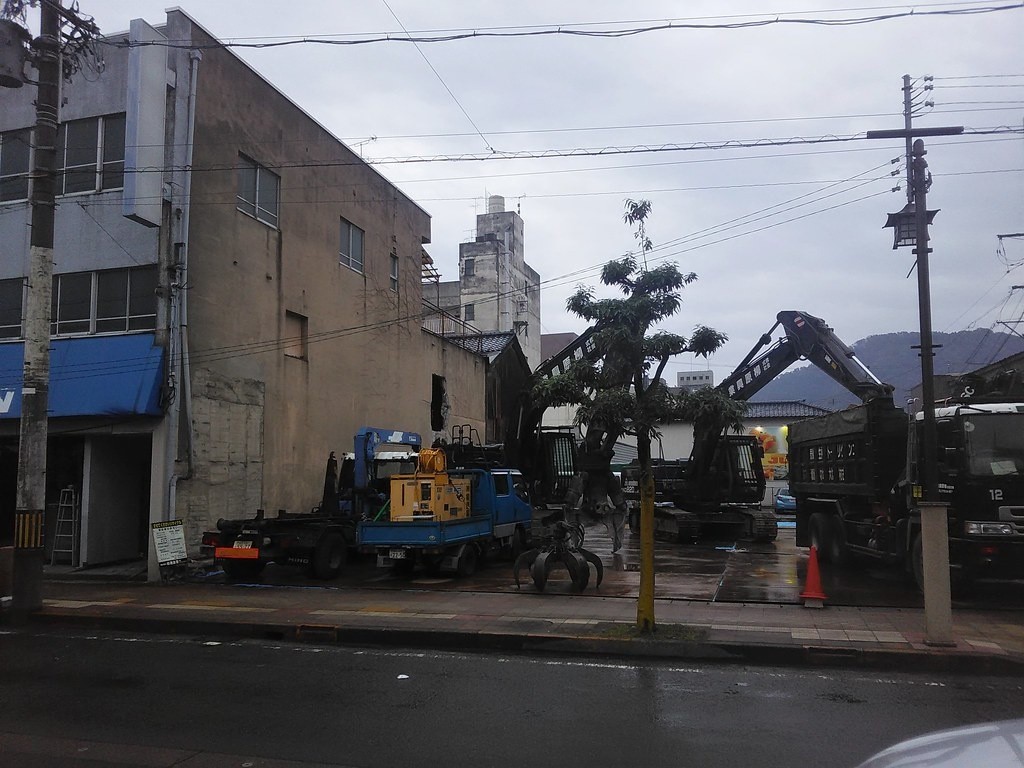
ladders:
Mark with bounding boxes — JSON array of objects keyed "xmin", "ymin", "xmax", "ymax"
[{"xmin": 50, "ymin": 485, "xmax": 80, "ymax": 568}]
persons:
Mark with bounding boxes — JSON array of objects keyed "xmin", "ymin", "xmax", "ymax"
[{"xmin": 511, "ymin": 474, "xmax": 567, "ymax": 513}]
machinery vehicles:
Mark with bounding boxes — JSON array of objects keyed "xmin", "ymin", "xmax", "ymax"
[
  {"xmin": 618, "ymin": 310, "xmax": 893, "ymax": 542},
  {"xmin": 200, "ymin": 423, "xmax": 535, "ymax": 588}
]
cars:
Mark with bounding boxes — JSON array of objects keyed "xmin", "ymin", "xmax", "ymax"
[{"xmin": 773, "ymin": 488, "xmax": 798, "ymax": 513}]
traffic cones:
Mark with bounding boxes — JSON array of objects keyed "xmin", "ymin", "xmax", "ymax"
[{"xmin": 800, "ymin": 547, "xmax": 829, "ymax": 601}]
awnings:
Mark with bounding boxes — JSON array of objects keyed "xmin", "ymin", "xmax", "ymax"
[{"xmin": 0, "ymin": 334, "xmax": 165, "ymax": 419}]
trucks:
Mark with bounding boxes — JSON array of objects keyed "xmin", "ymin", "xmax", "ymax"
[{"xmin": 785, "ymin": 399, "xmax": 1024, "ymax": 593}]
